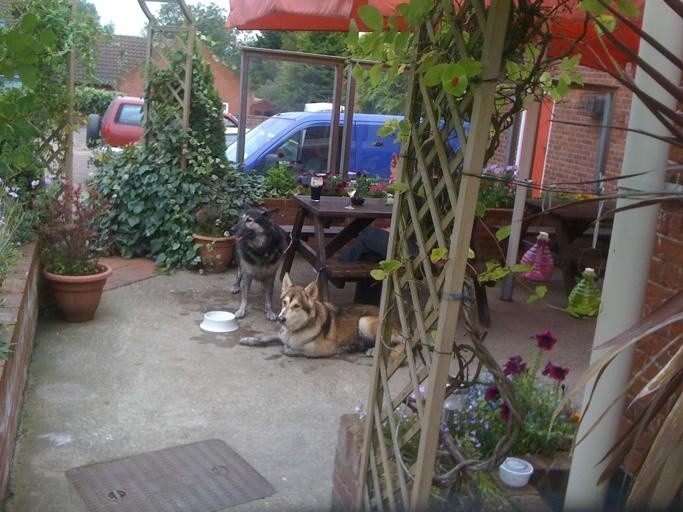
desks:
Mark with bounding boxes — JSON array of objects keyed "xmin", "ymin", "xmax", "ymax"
[
  {"xmin": 279, "ymin": 195, "xmax": 488, "ymax": 323},
  {"xmin": 525, "ymin": 199, "xmax": 615, "ymax": 297}
]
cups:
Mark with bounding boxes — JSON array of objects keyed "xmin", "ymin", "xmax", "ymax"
[{"xmin": 309, "ymin": 176, "xmax": 323, "ymax": 203}]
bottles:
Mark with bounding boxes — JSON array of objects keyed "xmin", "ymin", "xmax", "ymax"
[
  {"xmin": 567, "ymin": 268, "xmax": 602, "ymax": 318},
  {"xmin": 520, "ymin": 231, "xmax": 554, "ymax": 284}
]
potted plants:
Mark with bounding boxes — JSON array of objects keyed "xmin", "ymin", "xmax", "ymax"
[
  {"xmin": 44, "ymin": 178, "xmax": 112, "ymax": 322},
  {"xmin": 192, "ymin": 211, "xmax": 236, "ymax": 273}
]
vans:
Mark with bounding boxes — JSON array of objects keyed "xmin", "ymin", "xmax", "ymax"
[{"xmin": 225, "ymin": 111, "xmax": 469, "ymax": 184}]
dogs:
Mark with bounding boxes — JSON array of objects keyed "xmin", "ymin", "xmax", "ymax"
[
  {"xmin": 238, "ymin": 272, "xmax": 412, "ymax": 367},
  {"xmin": 230, "ymin": 202, "xmax": 291, "ymax": 321}
]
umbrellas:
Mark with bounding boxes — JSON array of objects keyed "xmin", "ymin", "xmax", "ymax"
[{"xmin": 222, "ymin": 0, "xmax": 648, "ymax": 82}]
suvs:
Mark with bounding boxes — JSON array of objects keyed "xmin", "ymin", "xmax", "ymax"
[{"xmin": 86, "ymin": 97, "xmax": 250, "ymax": 165}]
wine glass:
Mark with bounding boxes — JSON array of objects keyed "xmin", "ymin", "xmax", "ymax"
[{"xmin": 342, "ymin": 182, "xmax": 358, "ymax": 211}]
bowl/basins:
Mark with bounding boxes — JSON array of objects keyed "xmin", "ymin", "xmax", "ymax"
[
  {"xmin": 499, "ymin": 456, "xmax": 533, "ymax": 488},
  {"xmin": 199, "ymin": 310, "xmax": 238, "ymax": 333}
]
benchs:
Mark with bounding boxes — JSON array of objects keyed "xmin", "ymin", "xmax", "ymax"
[
  {"xmin": 578, "ymin": 259, "xmax": 606, "ymax": 275},
  {"xmin": 278, "ymin": 224, "xmax": 345, "ymax": 237},
  {"xmin": 493, "ymin": 224, "xmax": 612, "ymax": 240},
  {"xmin": 323, "ymin": 262, "xmax": 471, "ymax": 282}
]
